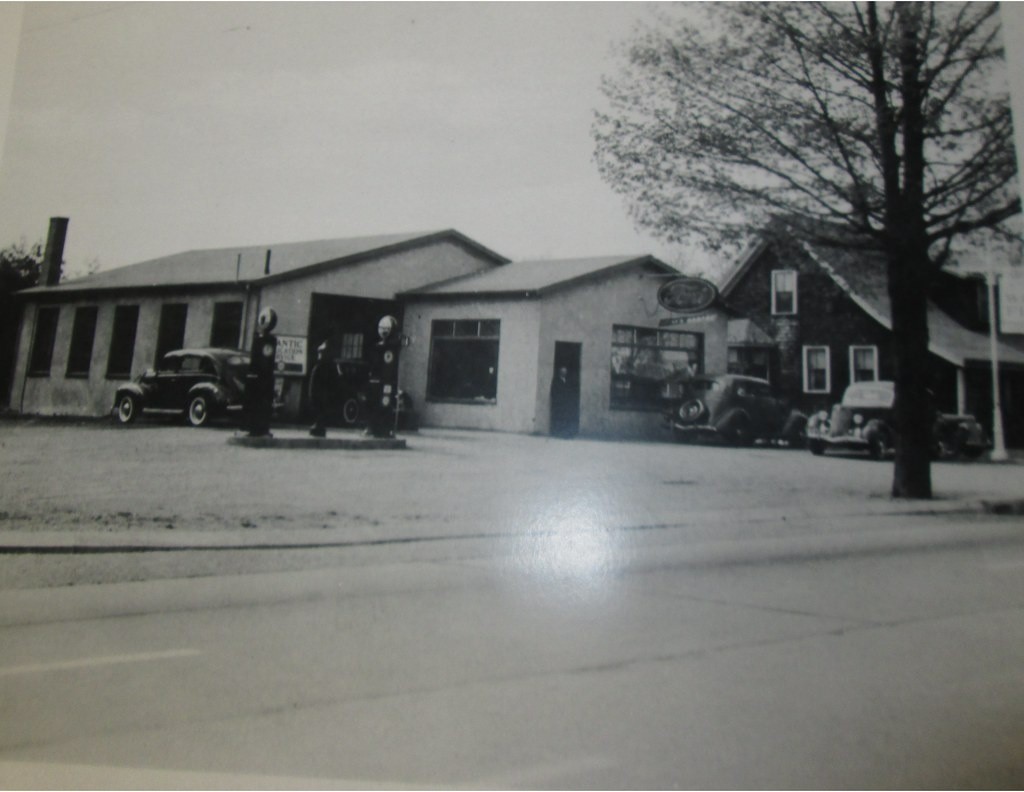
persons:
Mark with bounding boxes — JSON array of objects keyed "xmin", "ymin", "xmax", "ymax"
[{"xmin": 548, "ymin": 366, "xmax": 570, "ymax": 432}]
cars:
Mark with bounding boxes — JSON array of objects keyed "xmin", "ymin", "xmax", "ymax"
[
  {"xmin": 110, "ymin": 346, "xmax": 288, "ymax": 428},
  {"xmin": 657, "ymin": 371, "xmax": 808, "ymax": 448},
  {"xmin": 804, "ymin": 378, "xmax": 994, "ymax": 462},
  {"xmin": 330, "ymin": 356, "xmax": 414, "ymax": 430}
]
